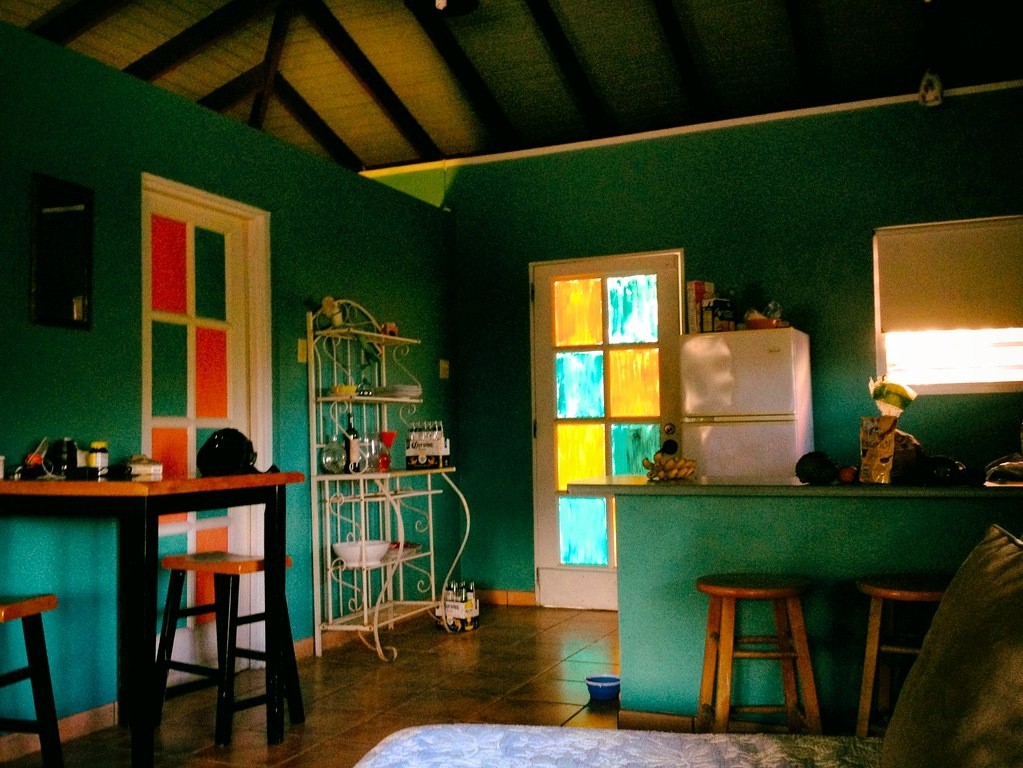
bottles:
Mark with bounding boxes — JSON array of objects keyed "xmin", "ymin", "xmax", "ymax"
[
  {"xmin": 343, "ymin": 412, "xmax": 360, "ymax": 473},
  {"xmin": 322, "ymin": 434, "xmax": 346, "ymax": 473},
  {"xmin": 443, "ymin": 580, "xmax": 475, "ymax": 602},
  {"xmin": 88, "ymin": 442, "xmax": 109, "ymax": 475},
  {"xmin": 406, "ymin": 420, "xmax": 443, "ymax": 441}
]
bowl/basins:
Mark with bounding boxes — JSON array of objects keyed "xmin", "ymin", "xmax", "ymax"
[
  {"xmin": 332, "ymin": 385, "xmax": 357, "ymax": 394},
  {"xmin": 585, "ymin": 674, "xmax": 620, "ymax": 702},
  {"xmin": 746, "ymin": 318, "xmax": 781, "ymax": 329},
  {"xmin": 333, "ymin": 540, "xmax": 390, "ymax": 567}
]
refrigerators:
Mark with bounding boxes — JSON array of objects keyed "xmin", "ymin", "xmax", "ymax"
[{"xmin": 677, "ymin": 327, "xmax": 815, "ymax": 481}]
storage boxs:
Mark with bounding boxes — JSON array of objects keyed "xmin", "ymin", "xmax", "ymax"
[
  {"xmin": 435, "ymin": 599, "xmax": 480, "ymax": 632},
  {"xmin": 406, "ymin": 438, "xmax": 450, "ymax": 470}
]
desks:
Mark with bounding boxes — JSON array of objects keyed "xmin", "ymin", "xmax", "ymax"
[{"xmin": 0, "ymin": 472, "xmax": 304, "ymax": 768}]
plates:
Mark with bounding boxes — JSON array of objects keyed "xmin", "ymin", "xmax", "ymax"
[{"xmin": 374, "ymin": 383, "xmax": 422, "ymax": 398}]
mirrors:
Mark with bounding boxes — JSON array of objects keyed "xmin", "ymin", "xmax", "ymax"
[{"xmin": 27, "ymin": 173, "xmax": 94, "ymax": 332}]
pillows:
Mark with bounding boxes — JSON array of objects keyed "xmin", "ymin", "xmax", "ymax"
[{"xmin": 883, "ymin": 520, "xmax": 1023, "ymax": 768}]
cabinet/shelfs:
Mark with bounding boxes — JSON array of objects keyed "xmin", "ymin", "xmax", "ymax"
[
  {"xmin": 306, "ymin": 300, "xmax": 471, "ymax": 664},
  {"xmin": 566, "ymin": 475, "xmax": 1023, "ymax": 735}
]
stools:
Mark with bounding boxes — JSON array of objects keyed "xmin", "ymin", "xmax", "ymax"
[
  {"xmin": 153, "ymin": 551, "xmax": 305, "ymax": 749},
  {"xmin": 0, "ymin": 593, "xmax": 63, "ymax": 768},
  {"xmin": 695, "ymin": 574, "xmax": 822, "ymax": 734},
  {"xmin": 855, "ymin": 577, "xmax": 948, "ymax": 737}
]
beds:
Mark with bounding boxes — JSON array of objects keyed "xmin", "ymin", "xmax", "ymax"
[{"xmin": 352, "ymin": 722, "xmax": 880, "ymax": 767}]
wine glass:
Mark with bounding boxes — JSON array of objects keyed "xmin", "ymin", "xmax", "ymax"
[{"xmin": 379, "ymin": 429, "xmax": 398, "ymax": 471}]
